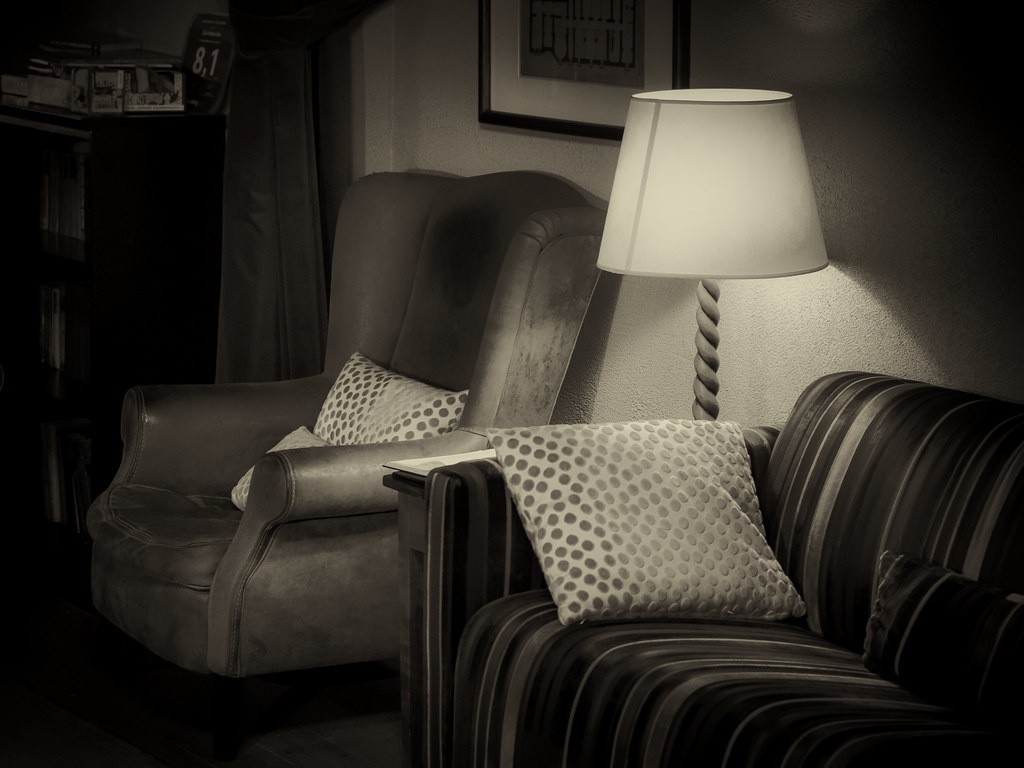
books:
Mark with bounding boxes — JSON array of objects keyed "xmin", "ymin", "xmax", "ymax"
[
  {"xmin": 40, "ymin": 149, "xmax": 86, "ymax": 240},
  {"xmin": 40, "ymin": 281, "xmax": 89, "ymax": 373},
  {"xmin": 40, "ymin": 417, "xmax": 92, "ymax": 535}
]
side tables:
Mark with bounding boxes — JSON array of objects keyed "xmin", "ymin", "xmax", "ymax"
[{"xmin": 381, "ymin": 472, "xmax": 428, "ymax": 768}]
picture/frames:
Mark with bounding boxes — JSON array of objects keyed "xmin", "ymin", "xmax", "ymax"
[{"xmin": 476, "ymin": 0, "xmax": 692, "ymax": 139}]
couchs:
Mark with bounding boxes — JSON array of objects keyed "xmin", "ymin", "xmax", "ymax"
[
  {"xmin": 428, "ymin": 372, "xmax": 1024, "ymax": 768},
  {"xmin": 84, "ymin": 168, "xmax": 613, "ymax": 761}
]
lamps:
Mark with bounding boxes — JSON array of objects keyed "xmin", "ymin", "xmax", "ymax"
[{"xmin": 596, "ymin": 88, "xmax": 832, "ymax": 428}]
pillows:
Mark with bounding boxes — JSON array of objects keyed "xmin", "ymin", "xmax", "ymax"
[
  {"xmin": 866, "ymin": 550, "xmax": 1024, "ymax": 724},
  {"xmin": 489, "ymin": 422, "xmax": 806, "ymax": 626},
  {"xmin": 230, "ymin": 424, "xmax": 334, "ymax": 512},
  {"xmin": 312, "ymin": 352, "xmax": 471, "ymax": 446}
]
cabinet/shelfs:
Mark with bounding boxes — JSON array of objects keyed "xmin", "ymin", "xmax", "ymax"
[{"xmin": 0, "ymin": 106, "xmax": 226, "ymax": 527}]
